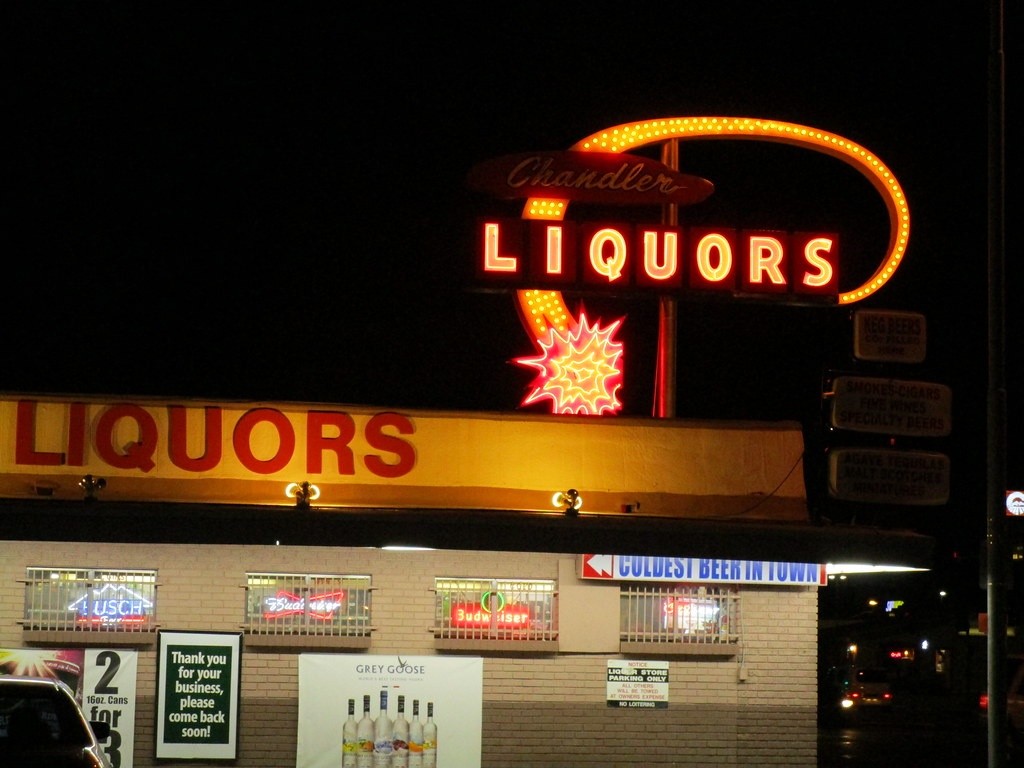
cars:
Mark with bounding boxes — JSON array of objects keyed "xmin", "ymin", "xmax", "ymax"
[
  {"xmin": 881, "ymin": 641, "xmax": 919, "ymax": 669},
  {"xmin": 827, "ymin": 664, "xmax": 893, "ymax": 713},
  {"xmin": 959, "ymin": 664, "xmax": 1024, "ymax": 738}
]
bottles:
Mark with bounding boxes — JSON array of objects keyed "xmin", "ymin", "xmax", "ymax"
[
  {"xmin": 381, "ymin": 686, "xmax": 388, "ymax": 691},
  {"xmin": 342, "ymin": 699, "xmax": 358, "ymax": 768},
  {"xmin": 357, "ymin": 695, "xmax": 374, "ymax": 768},
  {"xmin": 374, "ymin": 690, "xmax": 391, "ymax": 768},
  {"xmin": 422, "ymin": 703, "xmax": 437, "ymax": 768},
  {"xmin": 408, "ymin": 700, "xmax": 422, "ymax": 768},
  {"xmin": 392, "ymin": 686, "xmax": 400, "ymax": 722},
  {"xmin": 391, "ymin": 695, "xmax": 408, "ymax": 768}
]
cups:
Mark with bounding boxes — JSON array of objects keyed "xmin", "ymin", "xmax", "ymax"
[
  {"xmin": 935, "ymin": 647, "xmax": 951, "ymax": 673},
  {"xmin": 978, "ymin": 613, "xmax": 987, "ymax": 631}
]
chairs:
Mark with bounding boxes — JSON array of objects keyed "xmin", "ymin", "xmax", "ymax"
[{"xmin": 7, "ymin": 705, "xmax": 54, "ymax": 744}]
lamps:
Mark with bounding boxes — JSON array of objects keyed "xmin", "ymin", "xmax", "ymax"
[
  {"xmin": 80, "ymin": 474, "xmax": 106, "ymax": 502},
  {"xmin": 552, "ymin": 490, "xmax": 583, "ymax": 514},
  {"xmin": 285, "ymin": 480, "xmax": 320, "ymax": 506}
]
